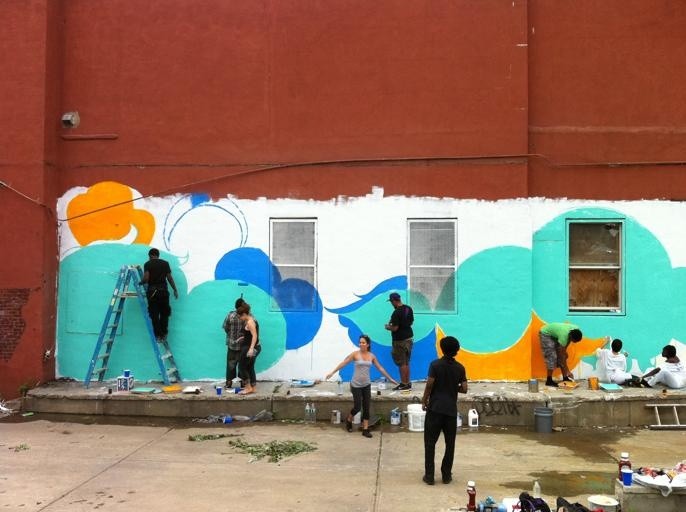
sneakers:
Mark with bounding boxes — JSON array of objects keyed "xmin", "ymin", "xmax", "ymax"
[
  {"xmin": 345, "ymin": 417, "xmax": 353, "ymax": 433},
  {"xmin": 393, "ymin": 381, "xmax": 412, "ymax": 392},
  {"xmin": 362, "ymin": 427, "xmax": 373, "ymax": 437}
]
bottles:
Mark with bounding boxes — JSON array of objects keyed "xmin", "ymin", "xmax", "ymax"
[
  {"xmin": 338, "ymin": 380, "xmax": 343, "ymax": 396},
  {"xmin": 304, "ymin": 402, "xmax": 317, "ymax": 423},
  {"xmin": 528, "ymin": 379, "xmax": 539, "ymax": 392},
  {"xmin": 330, "ymin": 409, "xmax": 342, "ymax": 425},
  {"xmin": 618, "ymin": 451, "xmax": 631, "ymax": 481},
  {"xmin": 381, "ymin": 377, "xmax": 388, "ymax": 389},
  {"xmin": 456, "ymin": 411, "xmax": 463, "ymax": 427},
  {"xmin": 465, "ymin": 481, "xmax": 477, "ymax": 509},
  {"xmin": 533, "ymin": 481, "xmax": 541, "ymax": 498}
]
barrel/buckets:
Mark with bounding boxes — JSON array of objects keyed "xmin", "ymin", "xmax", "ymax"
[
  {"xmin": 587, "ymin": 376, "xmax": 599, "ymax": 390},
  {"xmin": 407, "ymin": 403, "xmax": 427, "ymax": 432},
  {"xmin": 533, "ymin": 407, "xmax": 554, "ymax": 433},
  {"xmin": 467, "ymin": 408, "xmax": 479, "ymax": 428}
]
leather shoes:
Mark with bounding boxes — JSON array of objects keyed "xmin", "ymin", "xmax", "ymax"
[
  {"xmin": 442, "ymin": 475, "xmax": 453, "ymax": 484},
  {"xmin": 422, "ymin": 473, "xmax": 434, "ymax": 484}
]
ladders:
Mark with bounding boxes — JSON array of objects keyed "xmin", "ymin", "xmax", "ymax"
[
  {"xmin": 645, "ymin": 404, "xmax": 686, "ymax": 430},
  {"xmin": 83, "ymin": 265, "xmax": 181, "ymax": 388}
]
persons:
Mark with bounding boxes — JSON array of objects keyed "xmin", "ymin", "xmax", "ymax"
[
  {"xmin": 234, "ymin": 306, "xmax": 262, "ymax": 394},
  {"xmin": 138, "ymin": 247, "xmax": 179, "ymax": 344},
  {"xmin": 595, "ymin": 336, "xmax": 639, "ymax": 383},
  {"xmin": 420, "ymin": 335, "xmax": 467, "ymax": 485},
  {"xmin": 385, "ymin": 292, "xmax": 416, "ymax": 391},
  {"xmin": 539, "ymin": 320, "xmax": 584, "ymax": 387},
  {"xmin": 222, "ymin": 298, "xmax": 250, "ymax": 388},
  {"xmin": 325, "ymin": 335, "xmax": 398, "ymax": 437},
  {"xmin": 634, "ymin": 346, "xmax": 686, "ymax": 390}
]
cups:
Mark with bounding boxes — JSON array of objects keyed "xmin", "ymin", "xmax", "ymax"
[
  {"xmin": 123, "ymin": 370, "xmax": 130, "ymax": 376},
  {"xmin": 588, "ymin": 377, "xmax": 600, "ymax": 390},
  {"xmin": 621, "ymin": 468, "xmax": 633, "ymax": 486},
  {"xmin": 235, "ymin": 386, "xmax": 241, "ymax": 393},
  {"xmin": 390, "ymin": 411, "xmax": 402, "ymax": 424},
  {"xmin": 108, "ymin": 388, "xmax": 112, "ymax": 394},
  {"xmin": 216, "ymin": 386, "xmax": 222, "ymax": 394},
  {"xmin": 224, "ymin": 416, "xmax": 232, "ymax": 423}
]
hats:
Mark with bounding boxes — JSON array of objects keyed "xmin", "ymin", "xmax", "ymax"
[{"xmin": 385, "ymin": 293, "xmax": 401, "ymax": 301}]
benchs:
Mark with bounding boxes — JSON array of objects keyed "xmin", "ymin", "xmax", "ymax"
[{"xmin": 614, "ymin": 478, "xmax": 686, "ymax": 511}]
